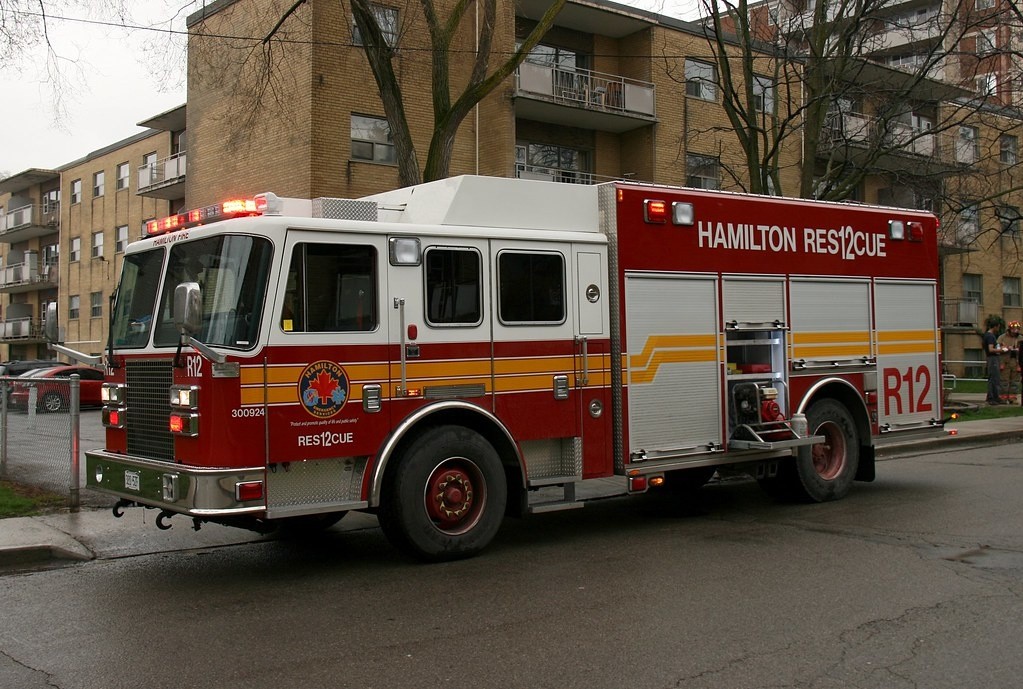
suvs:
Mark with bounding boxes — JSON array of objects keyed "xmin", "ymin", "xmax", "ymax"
[{"xmin": 0, "ymin": 360, "xmax": 72, "ymax": 405}]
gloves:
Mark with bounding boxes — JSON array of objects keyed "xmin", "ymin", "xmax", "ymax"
[{"xmin": 1002, "ymin": 347, "xmax": 1009, "ymax": 352}]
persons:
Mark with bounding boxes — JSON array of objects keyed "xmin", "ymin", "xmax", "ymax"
[
  {"xmin": 243, "ymin": 269, "xmax": 295, "ymax": 325},
  {"xmin": 982, "ymin": 317, "xmax": 1023, "ymax": 404}
]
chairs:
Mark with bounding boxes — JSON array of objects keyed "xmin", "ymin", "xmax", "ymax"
[{"xmin": 588, "ymin": 87, "xmax": 607, "ymax": 106}]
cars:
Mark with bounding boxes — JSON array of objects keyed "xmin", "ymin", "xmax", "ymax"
[
  {"xmin": 9, "ymin": 365, "xmax": 104, "ymax": 414},
  {"xmin": 6, "ymin": 367, "xmax": 52, "ymax": 400}
]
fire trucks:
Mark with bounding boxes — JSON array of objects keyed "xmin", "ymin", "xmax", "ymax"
[{"xmin": 81, "ymin": 176, "xmax": 958, "ymax": 554}]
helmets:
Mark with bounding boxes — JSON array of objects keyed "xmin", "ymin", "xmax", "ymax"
[{"xmin": 1008, "ymin": 321, "xmax": 1022, "ymax": 333}]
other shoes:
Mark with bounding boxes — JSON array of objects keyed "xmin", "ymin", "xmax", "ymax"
[
  {"xmin": 1010, "ymin": 399, "xmax": 1018, "ymax": 405},
  {"xmin": 997, "ymin": 398, "xmax": 1007, "ymax": 404},
  {"xmin": 985, "ymin": 400, "xmax": 999, "ymax": 405}
]
